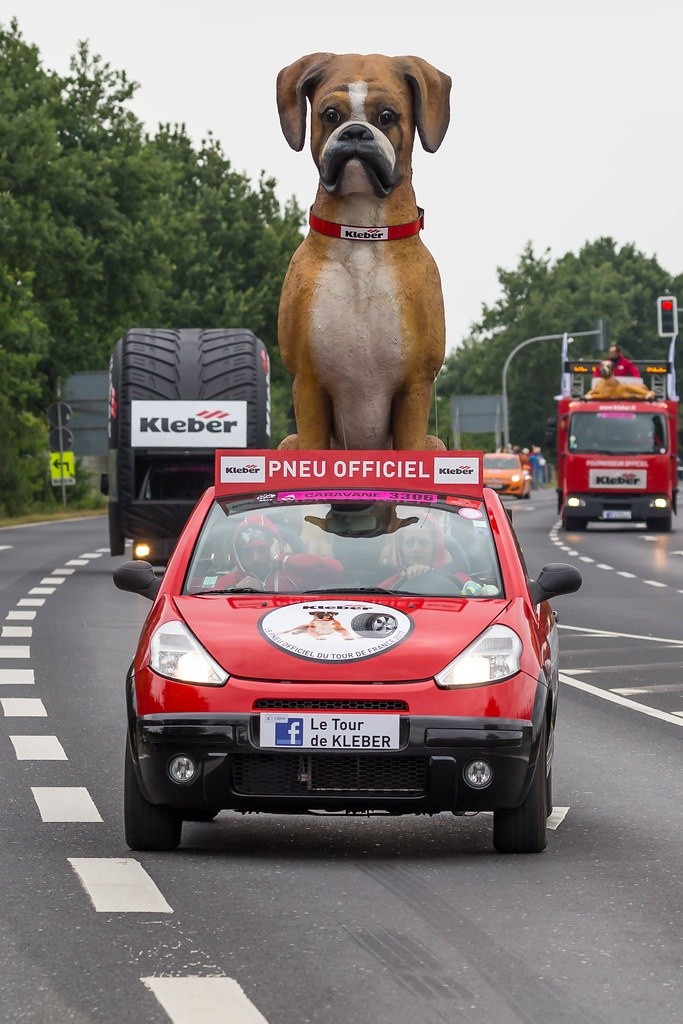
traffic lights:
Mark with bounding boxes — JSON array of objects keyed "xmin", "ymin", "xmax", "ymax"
[{"xmin": 656, "ymin": 296, "xmax": 679, "ymax": 338}]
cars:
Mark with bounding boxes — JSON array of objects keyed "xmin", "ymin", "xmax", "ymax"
[
  {"xmin": 483, "ymin": 452, "xmax": 533, "ymax": 500},
  {"xmin": 112, "ymin": 446, "xmax": 583, "ymax": 852}
]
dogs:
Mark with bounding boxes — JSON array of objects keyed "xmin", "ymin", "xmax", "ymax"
[{"xmin": 274, "ymin": 49, "xmax": 455, "ymax": 457}]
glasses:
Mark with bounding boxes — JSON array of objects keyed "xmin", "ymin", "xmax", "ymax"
[{"xmin": 239, "ymin": 533, "xmax": 269, "ymax": 545}]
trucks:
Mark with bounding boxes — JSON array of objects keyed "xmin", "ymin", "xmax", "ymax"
[{"xmin": 554, "ymin": 360, "xmax": 677, "ymax": 532}]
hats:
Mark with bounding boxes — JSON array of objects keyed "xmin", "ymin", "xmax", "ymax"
[{"xmin": 239, "ymin": 530, "xmax": 269, "ymax": 549}]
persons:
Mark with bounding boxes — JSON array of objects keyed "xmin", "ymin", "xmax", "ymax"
[
  {"xmin": 635, "ymin": 422, "xmax": 661, "ymax": 446},
  {"xmin": 214, "ymin": 516, "xmax": 342, "ymax": 593},
  {"xmin": 594, "ymin": 346, "xmax": 640, "ymax": 378},
  {"xmin": 376, "ymin": 514, "xmax": 472, "ymax": 591},
  {"xmin": 500, "ymin": 443, "xmax": 546, "ymax": 489}
]
